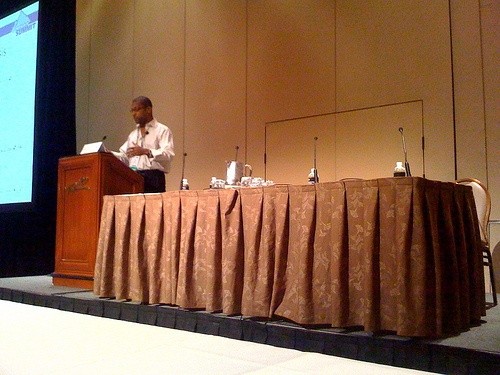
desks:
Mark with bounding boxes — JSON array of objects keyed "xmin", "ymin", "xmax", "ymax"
[{"xmin": 93, "ymin": 176, "xmax": 486, "ymax": 339}]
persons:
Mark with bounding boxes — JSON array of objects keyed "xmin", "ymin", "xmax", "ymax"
[{"xmin": 104, "ymin": 96, "xmax": 176, "ymax": 193}]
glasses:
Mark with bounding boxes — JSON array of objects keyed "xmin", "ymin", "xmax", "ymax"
[{"xmin": 132, "ymin": 107, "xmax": 145, "ymax": 113}]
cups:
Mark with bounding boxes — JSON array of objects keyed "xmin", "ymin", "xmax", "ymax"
[
  {"xmin": 241, "ymin": 177, "xmax": 273, "ymax": 187},
  {"xmin": 211, "ymin": 177, "xmax": 226, "ymax": 189}
]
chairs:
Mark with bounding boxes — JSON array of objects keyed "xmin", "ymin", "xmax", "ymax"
[{"xmin": 453, "ymin": 177, "xmax": 499, "ymax": 306}]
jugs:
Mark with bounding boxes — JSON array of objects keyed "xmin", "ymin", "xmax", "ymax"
[{"xmin": 226, "ymin": 161, "xmax": 243, "ymax": 185}]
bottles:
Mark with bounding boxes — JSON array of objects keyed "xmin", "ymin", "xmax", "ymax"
[
  {"xmin": 394, "ymin": 162, "xmax": 406, "ymax": 177},
  {"xmin": 308, "ymin": 169, "xmax": 320, "ymax": 184},
  {"xmin": 183, "ymin": 179, "xmax": 189, "ymax": 190}
]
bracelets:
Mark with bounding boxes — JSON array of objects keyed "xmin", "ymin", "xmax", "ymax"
[{"xmin": 146, "ymin": 150, "xmax": 152, "ymax": 156}]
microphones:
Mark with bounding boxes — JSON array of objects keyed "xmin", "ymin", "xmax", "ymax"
[
  {"xmin": 235, "ymin": 146, "xmax": 239, "ymax": 161},
  {"xmin": 314, "ymin": 136, "xmax": 318, "ymax": 182},
  {"xmin": 100, "ymin": 136, "xmax": 106, "ymax": 141},
  {"xmin": 145, "ymin": 131, "xmax": 149, "ymax": 134},
  {"xmin": 180, "ymin": 152, "xmax": 187, "ymax": 190},
  {"xmin": 398, "ymin": 127, "xmax": 411, "ymax": 176}
]
